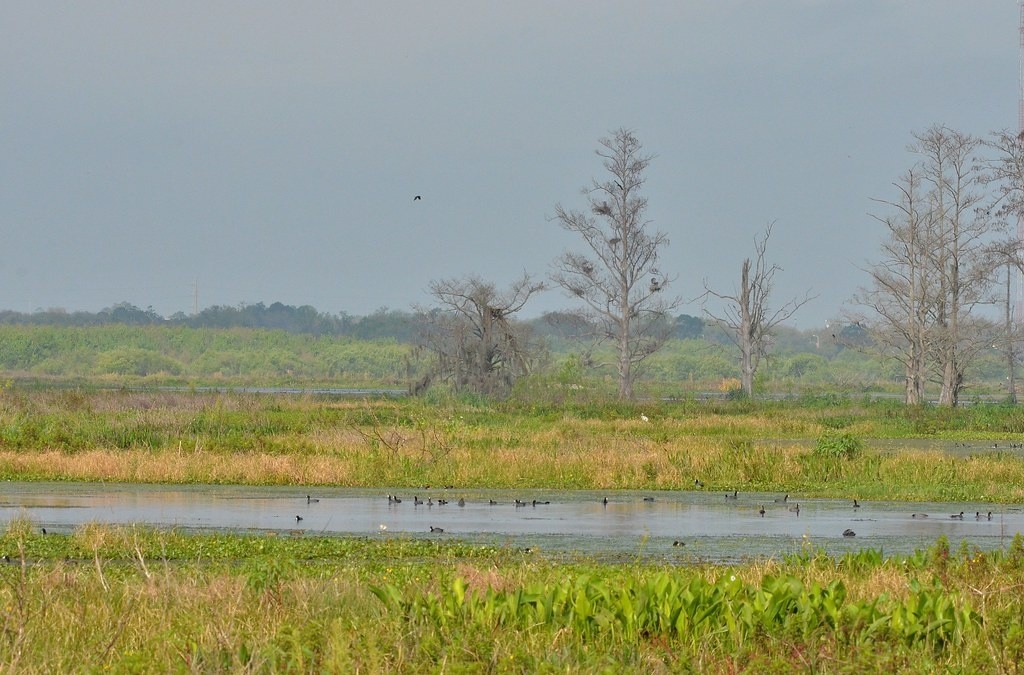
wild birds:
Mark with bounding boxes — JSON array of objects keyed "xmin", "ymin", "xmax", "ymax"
[
  {"xmin": 40, "ymin": 528, "xmax": 47, "ymax": 534},
  {"xmin": 694, "ymin": 479, "xmax": 859, "ymax": 537},
  {"xmin": 513, "ymin": 499, "xmax": 550, "ymax": 504},
  {"xmin": 643, "ymin": 497, "xmax": 654, "ymax": 502},
  {"xmin": 387, "ymin": 494, "xmax": 401, "ymax": 502},
  {"xmin": 438, "ymin": 500, "xmax": 447, "ymax": 504},
  {"xmin": 489, "ymin": 500, "xmax": 496, "ymax": 503},
  {"xmin": 306, "ymin": 495, "xmax": 319, "ymax": 502},
  {"xmin": 430, "ymin": 526, "xmax": 443, "ymax": 532},
  {"xmin": 604, "ymin": 497, "xmax": 607, "ymax": 503},
  {"xmin": 2, "ymin": 556, "xmax": 21, "ymax": 564},
  {"xmin": 427, "ymin": 497, "xmax": 433, "ymax": 505},
  {"xmin": 414, "ymin": 196, "xmax": 420, "ymax": 200},
  {"xmin": 674, "ymin": 541, "xmax": 684, "ymax": 546},
  {"xmin": 913, "ymin": 509, "xmax": 992, "ymax": 519},
  {"xmin": 458, "ymin": 498, "xmax": 465, "ymax": 506},
  {"xmin": 413, "ymin": 496, "xmax": 423, "ymax": 505},
  {"xmin": 955, "ymin": 439, "xmax": 1023, "ymax": 449},
  {"xmin": 295, "ymin": 516, "xmax": 303, "ymax": 521},
  {"xmin": 523, "ymin": 548, "xmax": 531, "ymax": 554}
]
